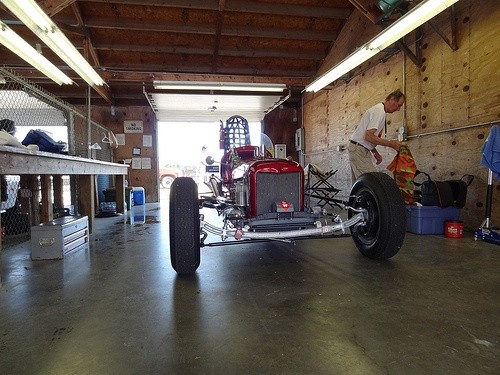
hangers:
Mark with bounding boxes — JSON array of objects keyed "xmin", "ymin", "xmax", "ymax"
[{"xmin": 102, "ymin": 132, "xmax": 113, "ymax": 144}]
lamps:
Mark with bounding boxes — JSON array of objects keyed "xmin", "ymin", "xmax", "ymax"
[
  {"xmin": 0, "ymin": 0, "xmax": 109, "ymax": 87},
  {"xmin": 152, "ymin": 80, "xmax": 287, "ymax": 92},
  {"xmin": 299, "ymin": 0, "xmax": 457, "ymax": 93}
]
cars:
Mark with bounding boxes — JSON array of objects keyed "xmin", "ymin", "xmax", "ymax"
[{"xmin": 169, "ymin": 132, "xmax": 407, "ymax": 276}]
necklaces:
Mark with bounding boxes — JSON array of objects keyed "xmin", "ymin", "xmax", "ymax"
[{"xmin": 382, "ymin": 102, "xmax": 387, "ymax": 139}]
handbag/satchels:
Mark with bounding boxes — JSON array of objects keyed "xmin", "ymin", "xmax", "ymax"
[
  {"xmin": 21, "ymin": 129, "xmax": 67, "ymax": 153},
  {"xmin": 413, "ymin": 169, "xmax": 474, "ymax": 208}
]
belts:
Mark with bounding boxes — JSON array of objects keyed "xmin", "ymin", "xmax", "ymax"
[{"xmin": 350, "ymin": 140, "xmax": 369, "ymax": 151}]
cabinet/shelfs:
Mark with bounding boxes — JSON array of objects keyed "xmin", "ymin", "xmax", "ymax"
[
  {"xmin": 105, "ymin": 186, "xmax": 133, "ymax": 212},
  {"xmin": 263, "ymin": 107, "xmax": 299, "ymax": 164}
]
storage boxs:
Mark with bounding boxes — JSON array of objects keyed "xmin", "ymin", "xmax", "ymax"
[
  {"xmin": 405, "ymin": 205, "xmax": 458, "ymax": 234},
  {"xmin": 134, "ymin": 187, "xmax": 146, "ymax": 205},
  {"xmin": 30, "ymin": 215, "xmax": 90, "ymax": 259},
  {"xmin": 21, "ymin": 129, "xmax": 66, "ymax": 153}
]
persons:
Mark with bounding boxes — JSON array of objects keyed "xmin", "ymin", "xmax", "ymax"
[{"xmin": 348, "ymin": 89, "xmax": 406, "ymax": 186}]
